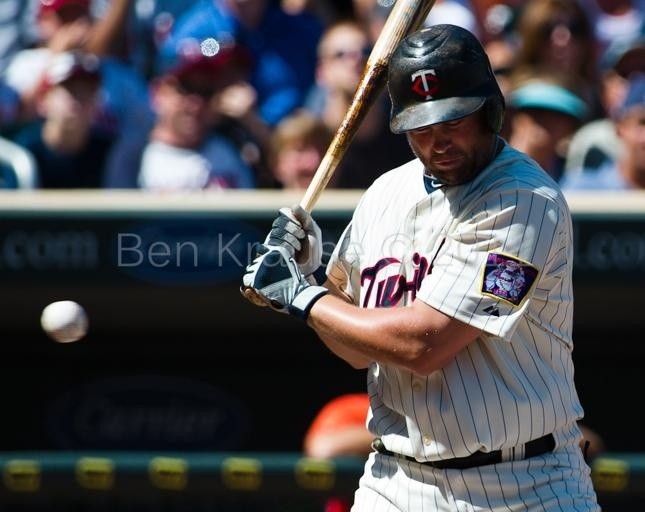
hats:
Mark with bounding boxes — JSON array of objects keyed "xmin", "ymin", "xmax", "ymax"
[
  {"xmin": 387, "ymin": 24, "xmax": 501, "ymax": 135},
  {"xmin": 46, "ymin": 49, "xmax": 98, "ymax": 88}
]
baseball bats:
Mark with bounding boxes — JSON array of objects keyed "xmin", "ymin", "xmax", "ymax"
[{"xmin": 247, "ymin": 0, "xmax": 435, "ymax": 310}]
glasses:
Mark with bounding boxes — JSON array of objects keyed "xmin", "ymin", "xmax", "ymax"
[{"xmin": 161, "ymin": 79, "xmax": 213, "ymax": 104}]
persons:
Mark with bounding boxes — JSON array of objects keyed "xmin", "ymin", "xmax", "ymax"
[
  {"xmin": 304, "ymin": 394, "xmax": 382, "ymax": 512},
  {"xmin": 238, "ymin": 24, "xmax": 602, "ymax": 512},
  {"xmin": 0, "ymin": 0, "xmax": 645, "ymax": 193}
]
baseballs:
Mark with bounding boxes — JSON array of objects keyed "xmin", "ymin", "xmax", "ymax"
[{"xmin": 41, "ymin": 300, "xmax": 86, "ymax": 344}]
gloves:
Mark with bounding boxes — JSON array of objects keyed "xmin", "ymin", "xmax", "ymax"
[
  {"xmin": 242, "ymin": 243, "xmax": 330, "ymax": 325},
  {"xmin": 263, "ymin": 204, "xmax": 328, "ymax": 286}
]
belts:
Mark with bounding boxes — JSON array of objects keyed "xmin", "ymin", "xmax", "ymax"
[{"xmin": 377, "ymin": 433, "xmax": 556, "ymax": 470}]
